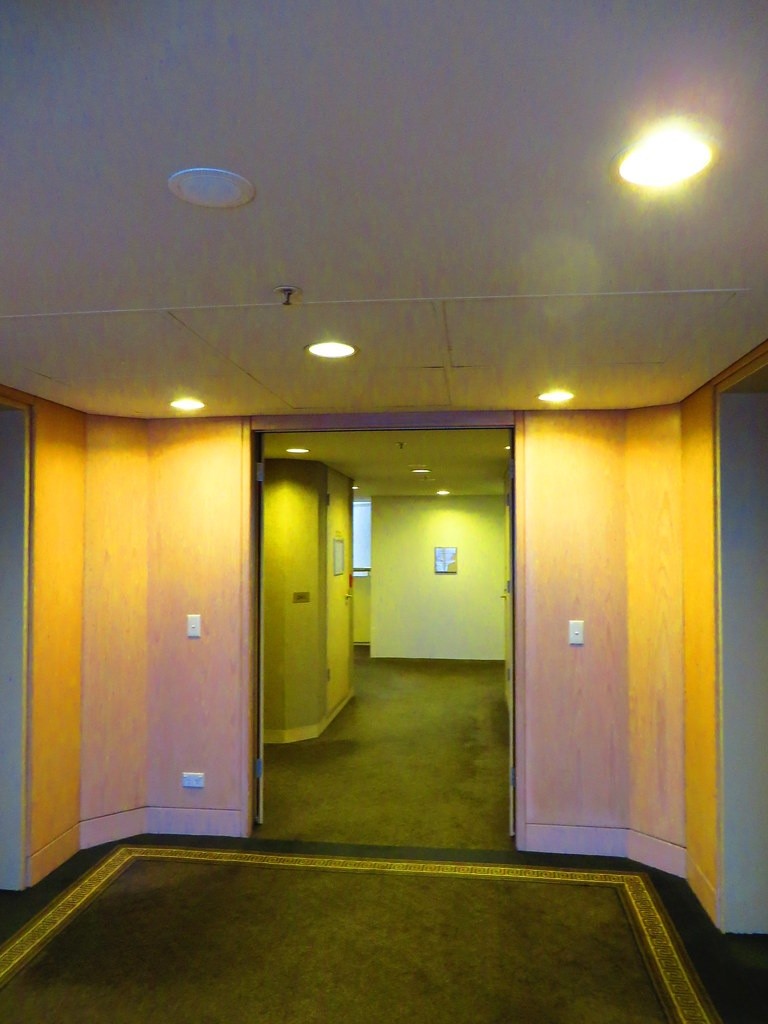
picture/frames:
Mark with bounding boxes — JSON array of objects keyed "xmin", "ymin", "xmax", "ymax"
[
  {"xmin": 333, "ymin": 537, "xmax": 345, "ymax": 576},
  {"xmin": 434, "ymin": 547, "xmax": 457, "ymax": 573}
]
